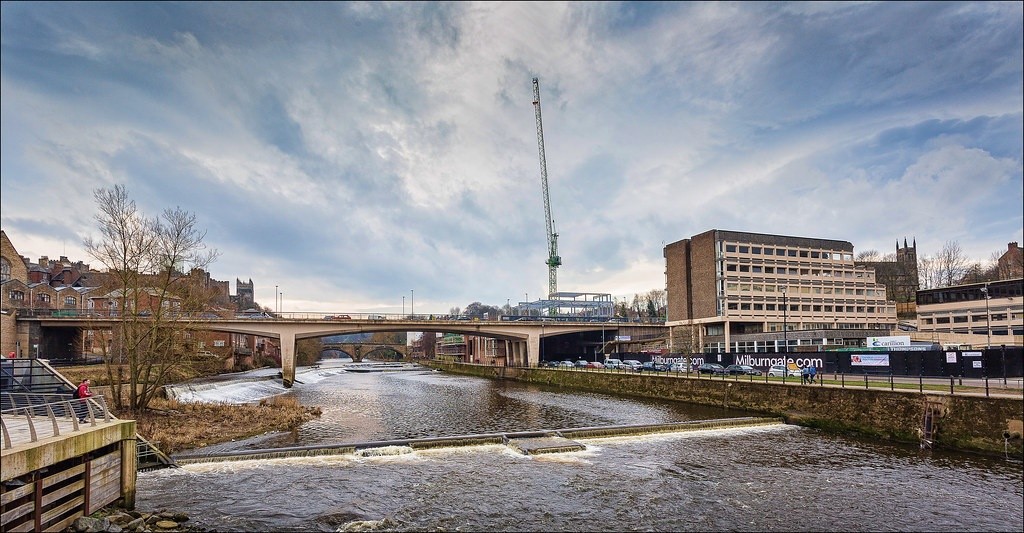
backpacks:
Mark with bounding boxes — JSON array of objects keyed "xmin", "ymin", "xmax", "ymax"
[{"xmin": 73, "ymin": 385, "xmax": 84, "ymax": 399}]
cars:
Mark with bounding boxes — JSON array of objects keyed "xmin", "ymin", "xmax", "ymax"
[
  {"xmin": 697, "ymin": 363, "xmax": 725, "ymax": 374},
  {"xmin": 559, "ymin": 361, "xmax": 576, "ymax": 367},
  {"xmin": 135, "ymin": 309, "xmax": 272, "ymax": 320},
  {"xmin": 539, "ymin": 360, "xmax": 559, "ymax": 368},
  {"xmin": 671, "ymin": 363, "xmax": 694, "ymax": 373},
  {"xmin": 574, "ymin": 360, "xmax": 588, "ymax": 368},
  {"xmin": 587, "ymin": 362, "xmax": 605, "ymax": 370},
  {"xmin": 458, "ymin": 315, "xmax": 473, "ymax": 321},
  {"xmin": 643, "ymin": 362, "xmax": 672, "ymax": 371}
]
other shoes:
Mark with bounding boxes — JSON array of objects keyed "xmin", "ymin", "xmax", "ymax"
[{"xmin": 79, "ymin": 419, "xmax": 87, "ymax": 424}]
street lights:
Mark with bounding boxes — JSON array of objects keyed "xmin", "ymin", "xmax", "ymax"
[
  {"xmin": 403, "ymin": 296, "xmax": 405, "ymax": 320},
  {"xmin": 33, "ymin": 344, "xmax": 39, "ymax": 359},
  {"xmin": 981, "ymin": 287, "xmax": 991, "ymax": 350},
  {"xmin": 30, "ymin": 288, "xmax": 33, "ymax": 309},
  {"xmin": 542, "ymin": 319, "xmax": 545, "ymax": 361},
  {"xmin": 411, "ymin": 289, "xmax": 415, "ymax": 320},
  {"xmin": 616, "ymin": 316, "xmax": 621, "ymax": 358},
  {"xmin": 280, "ymin": 292, "xmax": 283, "ymax": 317},
  {"xmin": 782, "ymin": 285, "xmax": 789, "ymax": 378},
  {"xmin": 603, "ymin": 318, "xmax": 610, "ymax": 358},
  {"xmin": 276, "ymin": 285, "xmax": 279, "ymax": 315}
]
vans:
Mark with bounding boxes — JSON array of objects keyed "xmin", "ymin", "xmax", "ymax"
[
  {"xmin": 622, "ymin": 360, "xmax": 644, "ymax": 372},
  {"xmin": 368, "ymin": 315, "xmax": 388, "ymax": 321},
  {"xmin": 604, "ymin": 359, "xmax": 624, "ymax": 370}
]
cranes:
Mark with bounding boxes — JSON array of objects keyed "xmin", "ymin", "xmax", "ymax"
[{"xmin": 531, "ymin": 76, "xmax": 573, "ymax": 320}]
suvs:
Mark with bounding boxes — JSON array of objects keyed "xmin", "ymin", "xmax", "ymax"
[
  {"xmin": 324, "ymin": 314, "xmax": 352, "ymax": 321},
  {"xmin": 768, "ymin": 364, "xmax": 805, "ymax": 379},
  {"xmin": 730, "ymin": 365, "xmax": 763, "ymax": 376}
]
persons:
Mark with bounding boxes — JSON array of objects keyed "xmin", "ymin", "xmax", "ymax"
[
  {"xmin": 653, "ymin": 361, "xmax": 656, "ymax": 373},
  {"xmin": 802, "ymin": 365, "xmax": 811, "ymax": 385},
  {"xmin": 810, "ymin": 366, "xmax": 817, "ymax": 385},
  {"xmin": 78, "ymin": 379, "xmax": 93, "ymax": 424},
  {"xmin": 851, "ymin": 356, "xmax": 859, "ymax": 362}
]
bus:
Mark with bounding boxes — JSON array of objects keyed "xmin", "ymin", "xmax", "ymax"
[
  {"xmin": 942, "ymin": 343, "xmax": 973, "ymax": 351},
  {"xmin": 497, "ymin": 315, "xmax": 629, "ymax": 321},
  {"xmin": 817, "ymin": 342, "xmax": 944, "ymax": 351}
]
